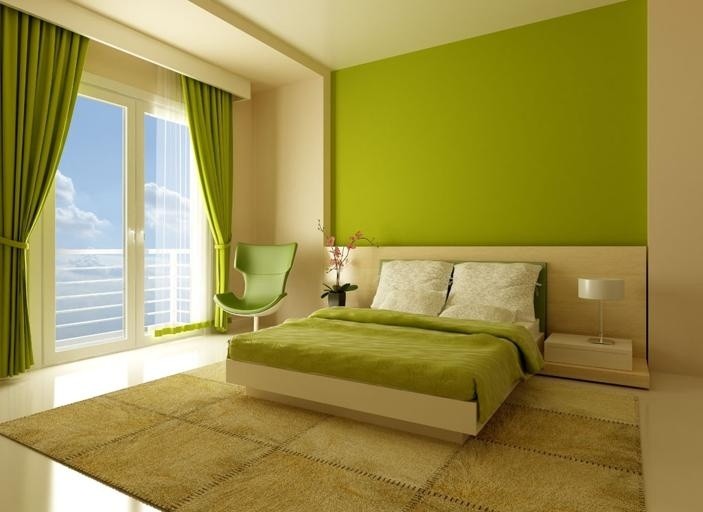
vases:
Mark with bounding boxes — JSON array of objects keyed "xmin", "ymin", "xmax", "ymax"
[{"xmin": 325, "ymin": 289, "xmax": 348, "ymax": 309}]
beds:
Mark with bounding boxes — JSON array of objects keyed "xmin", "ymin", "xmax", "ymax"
[{"xmin": 221, "ymin": 257, "xmax": 554, "ymax": 445}]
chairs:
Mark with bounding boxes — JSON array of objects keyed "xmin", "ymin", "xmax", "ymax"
[{"xmin": 212, "ymin": 241, "xmax": 299, "ymax": 335}]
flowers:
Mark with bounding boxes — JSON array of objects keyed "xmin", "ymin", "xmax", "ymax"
[{"xmin": 311, "ymin": 218, "xmax": 378, "ymax": 298}]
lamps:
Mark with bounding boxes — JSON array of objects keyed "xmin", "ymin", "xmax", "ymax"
[{"xmin": 569, "ymin": 274, "xmax": 627, "ymax": 346}]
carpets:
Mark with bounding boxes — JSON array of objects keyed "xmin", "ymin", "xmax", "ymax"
[{"xmin": 0, "ymin": 357, "xmax": 647, "ymax": 512}]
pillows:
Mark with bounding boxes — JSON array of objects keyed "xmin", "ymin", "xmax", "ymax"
[
  {"xmin": 436, "ymin": 259, "xmax": 546, "ymax": 322},
  {"xmin": 367, "ymin": 260, "xmax": 455, "ymax": 318}
]
